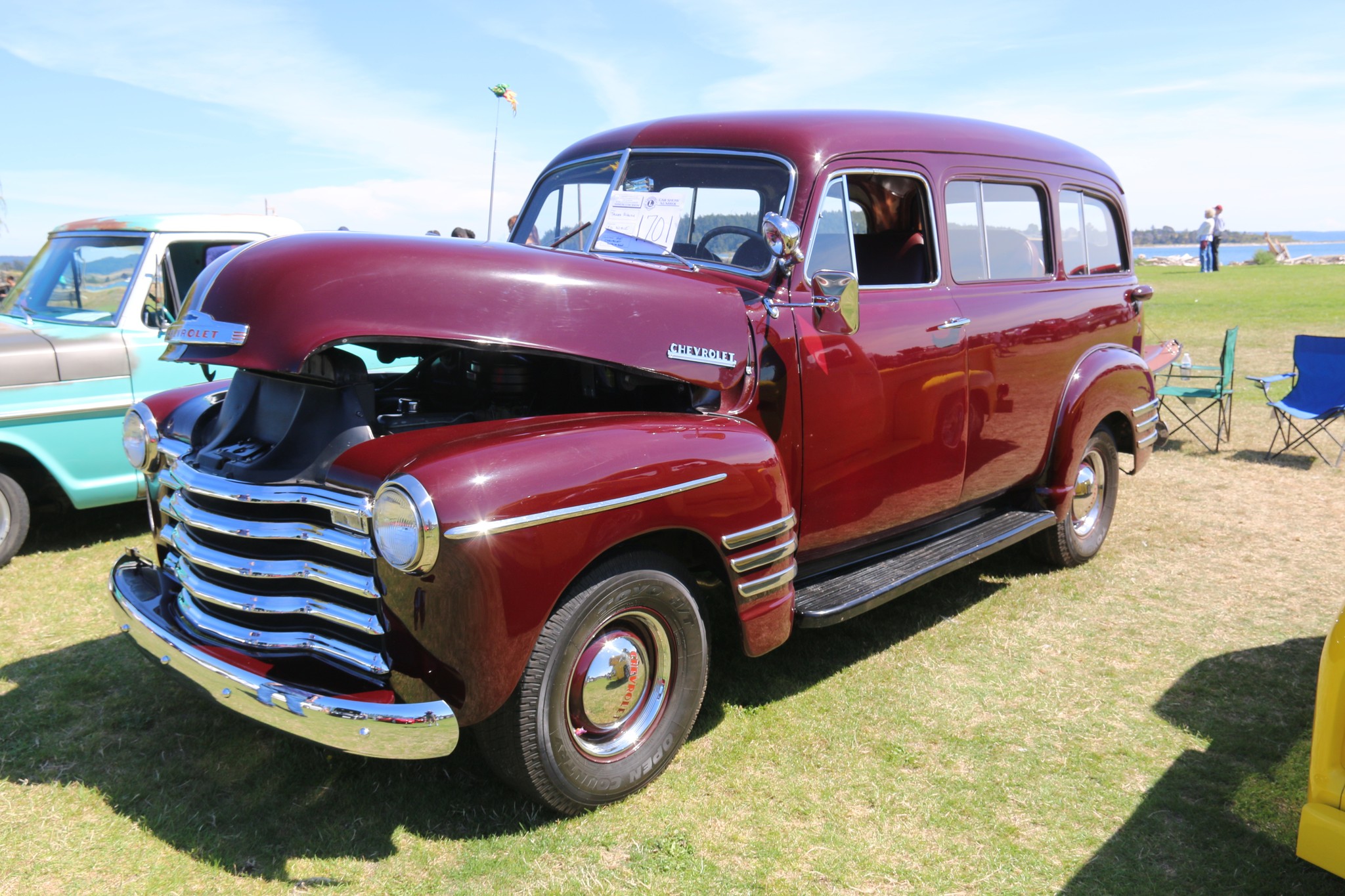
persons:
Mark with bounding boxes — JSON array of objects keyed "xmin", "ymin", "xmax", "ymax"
[
  {"xmin": 1198, "ymin": 206, "xmax": 1223, "ymax": 273},
  {"xmin": 337, "ymin": 214, "xmax": 542, "ymax": 246},
  {"xmin": 0, "ymin": 276, "xmax": 16, "ymax": 297}
]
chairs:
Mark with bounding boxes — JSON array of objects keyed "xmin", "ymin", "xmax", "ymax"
[
  {"xmin": 1245, "ymin": 335, "xmax": 1345, "ymax": 467},
  {"xmin": 1154, "ymin": 325, "xmax": 1239, "ymax": 455},
  {"xmin": 854, "ymin": 227, "xmax": 926, "ymax": 283}
]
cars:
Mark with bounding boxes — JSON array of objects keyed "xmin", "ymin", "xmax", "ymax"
[
  {"xmin": 109, "ymin": 105, "xmax": 1183, "ymax": 818},
  {"xmin": 0, "ymin": 214, "xmax": 311, "ymax": 566}
]
